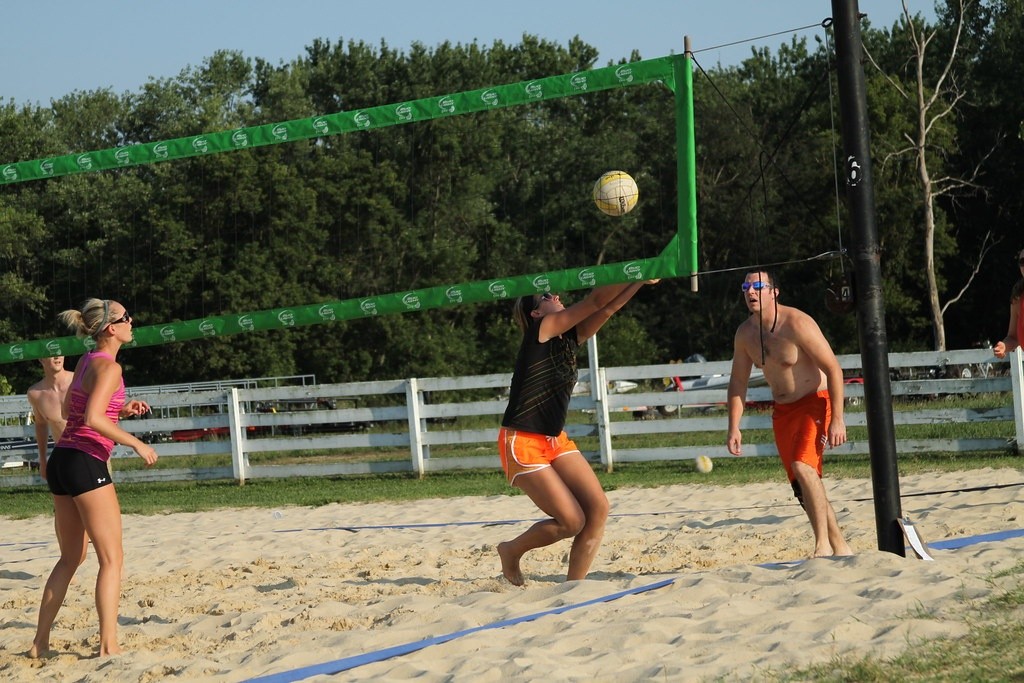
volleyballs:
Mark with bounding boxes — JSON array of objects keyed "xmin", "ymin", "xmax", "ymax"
[
  {"xmin": 593, "ymin": 170, "xmax": 639, "ymax": 217},
  {"xmin": 695, "ymin": 455, "xmax": 713, "ymax": 474}
]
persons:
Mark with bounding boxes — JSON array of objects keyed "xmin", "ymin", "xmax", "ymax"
[
  {"xmin": 993, "ymin": 249, "xmax": 1024, "ymax": 359},
  {"xmin": 496, "ymin": 278, "xmax": 662, "ymax": 586},
  {"xmin": 727, "ymin": 267, "xmax": 854, "ymax": 559},
  {"xmin": 27, "ymin": 299, "xmax": 159, "ymax": 660}
]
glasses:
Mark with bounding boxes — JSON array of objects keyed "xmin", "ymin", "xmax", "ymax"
[
  {"xmin": 102, "ymin": 310, "xmax": 130, "ymax": 333},
  {"xmin": 741, "ymin": 281, "xmax": 772, "ymax": 292},
  {"xmin": 1018, "ymin": 257, "xmax": 1024, "ymax": 266},
  {"xmin": 532, "ymin": 292, "xmax": 553, "ymax": 310}
]
